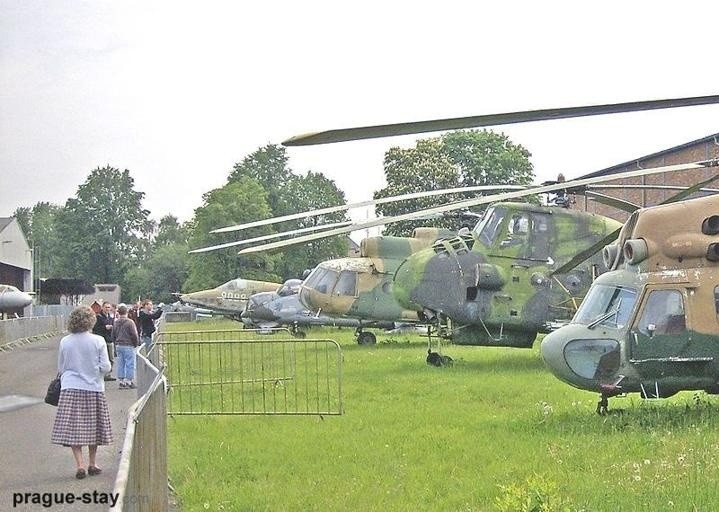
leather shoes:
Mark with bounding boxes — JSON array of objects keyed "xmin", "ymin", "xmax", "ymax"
[
  {"xmin": 88, "ymin": 466, "xmax": 102, "ymax": 475},
  {"xmin": 76, "ymin": 468, "xmax": 86, "ymax": 479}
]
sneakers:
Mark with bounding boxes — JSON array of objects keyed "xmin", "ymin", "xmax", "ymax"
[{"xmin": 104, "ymin": 375, "xmax": 137, "ymax": 390}]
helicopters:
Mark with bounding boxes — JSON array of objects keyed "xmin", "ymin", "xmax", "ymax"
[{"xmin": 0, "ymin": 283, "xmax": 36, "ymax": 320}]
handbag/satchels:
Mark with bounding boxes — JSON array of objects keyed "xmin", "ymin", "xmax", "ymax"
[{"xmin": 45, "ymin": 372, "xmax": 61, "ymax": 406}]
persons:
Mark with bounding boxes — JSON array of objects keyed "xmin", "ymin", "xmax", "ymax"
[{"xmin": 49, "ymin": 298, "xmax": 165, "ymax": 479}]
